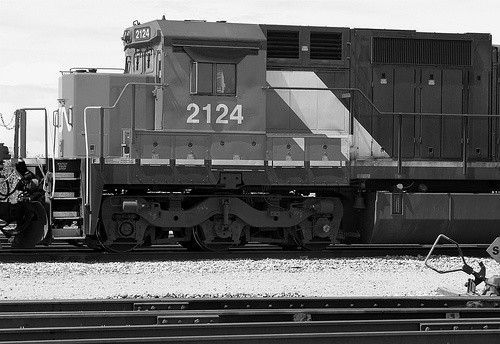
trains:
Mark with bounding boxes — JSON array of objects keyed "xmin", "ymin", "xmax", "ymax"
[{"xmin": 1, "ymin": 16, "xmax": 498, "ymax": 253}]
